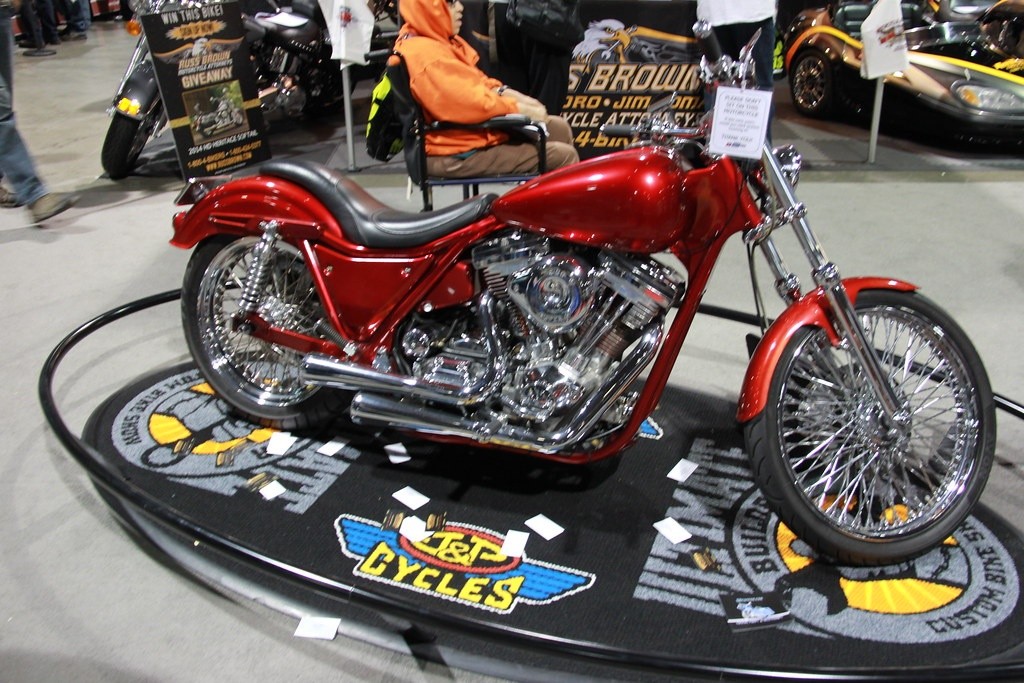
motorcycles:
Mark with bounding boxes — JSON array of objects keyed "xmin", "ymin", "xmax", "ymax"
[
  {"xmin": 165, "ymin": 16, "xmax": 999, "ymax": 569},
  {"xmin": 190, "ymin": 85, "xmax": 243, "ymax": 139},
  {"xmin": 782, "ymin": 0, "xmax": 1024, "ymax": 152},
  {"xmin": 100, "ymin": 0, "xmax": 367, "ymax": 181}
]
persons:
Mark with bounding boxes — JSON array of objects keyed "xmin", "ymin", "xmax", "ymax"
[
  {"xmin": 14, "ymin": 0, "xmax": 89, "ymax": 49},
  {"xmin": 386, "ymin": 0, "xmax": 580, "ymax": 182},
  {"xmin": 0, "ymin": 0, "xmax": 79, "ymax": 224},
  {"xmin": 697, "ymin": 0, "xmax": 779, "ymax": 144}
]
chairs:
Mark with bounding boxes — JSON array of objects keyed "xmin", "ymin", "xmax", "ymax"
[
  {"xmin": 835, "ymin": 4, "xmax": 870, "ymax": 41},
  {"xmin": 902, "ymin": 3, "xmax": 923, "ymax": 30},
  {"xmin": 383, "ymin": 50, "xmax": 549, "ymax": 213}
]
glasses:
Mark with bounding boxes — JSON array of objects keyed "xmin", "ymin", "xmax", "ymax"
[{"xmin": 445, "ymin": 0, "xmax": 457, "ymax": 4}]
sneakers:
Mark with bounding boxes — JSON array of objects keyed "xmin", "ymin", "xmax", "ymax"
[
  {"xmin": 0, "ymin": 185, "xmax": 24, "ymax": 208},
  {"xmin": 31, "ymin": 192, "xmax": 82, "ymax": 223}
]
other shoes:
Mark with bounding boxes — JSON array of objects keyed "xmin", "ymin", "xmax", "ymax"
[
  {"xmin": 92, "ymin": 13, "xmax": 113, "ymax": 22},
  {"xmin": 42, "ymin": 33, "xmax": 62, "ymax": 44},
  {"xmin": 19, "ymin": 38, "xmax": 46, "ymax": 49},
  {"xmin": 59, "ymin": 26, "xmax": 88, "ymax": 40},
  {"xmin": 121, "ymin": 10, "xmax": 133, "ymax": 22}
]
